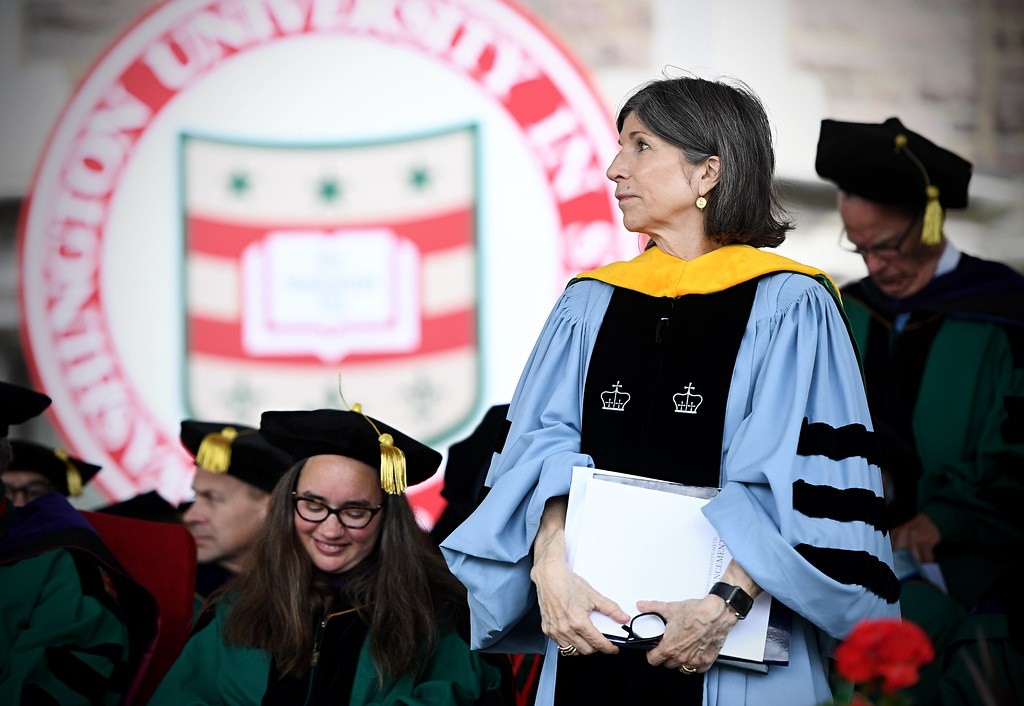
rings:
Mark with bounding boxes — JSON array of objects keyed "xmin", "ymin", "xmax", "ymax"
[
  {"xmin": 679, "ymin": 665, "xmax": 696, "ymax": 674},
  {"xmin": 558, "ymin": 645, "xmax": 576, "ymax": 656}
]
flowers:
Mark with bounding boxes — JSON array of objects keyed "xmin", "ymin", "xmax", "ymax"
[{"xmin": 819, "ymin": 616, "xmax": 937, "ymax": 706}]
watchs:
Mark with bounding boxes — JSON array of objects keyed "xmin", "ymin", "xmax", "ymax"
[{"xmin": 709, "ymin": 582, "xmax": 754, "ymax": 620}]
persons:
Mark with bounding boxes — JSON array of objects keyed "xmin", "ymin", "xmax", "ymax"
[
  {"xmin": 0, "ymin": 381, "xmax": 157, "ymax": 706},
  {"xmin": 181, "ymin": 419, "xmax": 272, "ymax": 637},
  {"xmin": 0, "ymin": 439, "xmax": 102, "ymax": 506},
  {"xmin": 815, "ymin": 117, "xmax": 1024, "ymax": 706},
  {"xmin": 144, "ymin": 405, "xmax": 514, "ymax": 706},
  {"xmin": 437, "ymin": 78, "xmax": 904, "ymax": 706}
]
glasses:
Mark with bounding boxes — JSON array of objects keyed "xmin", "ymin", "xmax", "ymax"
[
  {"xmin": 4, "ymin": 479, "xmax": 47, "ymax": 504},
  {"xmin": 292, "ymin": 492, "xmax": 387, "ymax": 530},
  {"xmin": 836, "ymin": 220, "xmax": 915, "ymax": 255},
  {"xmin": 599, "ymin": 612, "xmax": 667, "ymax": 648}
]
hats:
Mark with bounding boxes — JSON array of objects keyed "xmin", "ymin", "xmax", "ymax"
[
  {"xmin": 259, "ymin": 404, "xmax": 443, "ymax": 497},
  {"xmin": 816, "ymin": 116, "xmax": 976, "ymax": 248},
  {"xmin": 2, "ymin": 440, "xmax": 102, "ymax": 498},
  {"xmin": 180, "ymin": 419, "xmax": 265, "ymax": 488},
  {"xmin": 1, "ymin": 380, "xmax": 52, "ymax": 436}
]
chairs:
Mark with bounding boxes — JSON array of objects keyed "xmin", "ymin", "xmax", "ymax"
[{"xmin": 80, "ymin": 510, "xmax": 199, "ymax": 705}]
